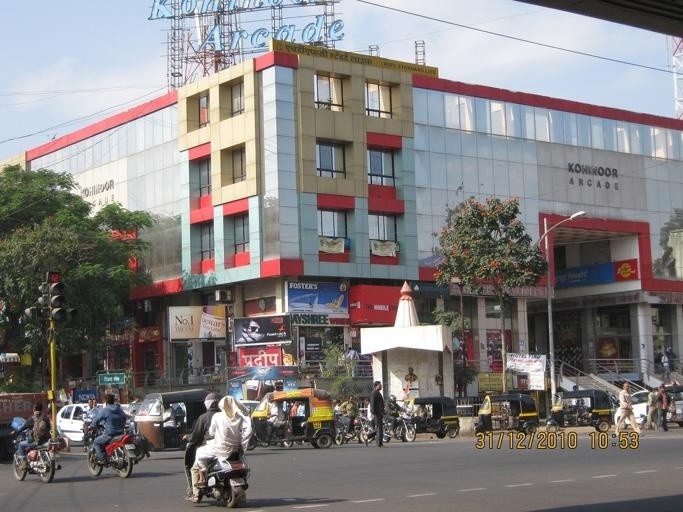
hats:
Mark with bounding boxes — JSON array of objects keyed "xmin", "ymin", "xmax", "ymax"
[{"xmin": 205, "ymin": 393, "xmax": 219, "ymax": 400}]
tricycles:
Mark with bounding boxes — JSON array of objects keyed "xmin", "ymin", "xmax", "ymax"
[
  {"xmin": 403, "ymin": 395, "xmax": 459, "ymax": 439},
  {"xmin": 474, "ymin": 394, "xmax": 539, "ymax": 437},
  {"xmin": 130, "ymin": 388, "xmax": 214, "ymax": 461},
  {"xmin": 544, "ymin": 389, "xmax": 612, "ymax": 435},
  {"xmin": 248, "ymin": 387, "xmax": 335, "ymax": 451}
]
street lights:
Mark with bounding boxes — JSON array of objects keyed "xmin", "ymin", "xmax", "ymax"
[{"xmin": 538, "ymin": 211, "xmax": 585, "ymax": 411}]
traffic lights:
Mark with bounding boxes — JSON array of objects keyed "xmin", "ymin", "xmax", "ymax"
[
  {"xmin": 38, "ymin": 282, "xmax": 49, "ymax": 317},
  {"xmin": 51, "ymin": 283, "xmax": 65, "ymax": 323},
  {"xmin": 65, "ymin": 309, "xmax": 79, "ymax": 333},
  {"xmin": 47, "ymin": 272, "xmax": 60, "ymax": 282}
]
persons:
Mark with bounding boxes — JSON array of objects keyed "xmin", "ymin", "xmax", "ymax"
[
  {"xmin": 346, "ymin": 346, "xmax": 360, "ymax": 378},
  {"xmin": 155, "ymin": 400, "xmax": 177, "ymax": 438},
  {"xmin": 266, "ymin": 400, "xmax": 279, "ymax": 425},
  {"xmin": 668, "ymin": 346, "xmax": 676, "ymax": 371},
  {"xmin": 182, "ymin": 391, "xmax": 222, "ymax": 501},
  {"xmin": 364, "ymin": 381, "xmax": 387, "ymax": 450},
  {"xmin": 238, "ymin": 317, "xmax": 271, "ymax": 342},
  {"xmin": 334, "ymin": 395, "xmax": 431, "ymax": 438},
  {"xmin": 12, "ymin": 400, "xmax": 50, "ymax": 463},
  {"xmin": 193, "ymin": 395, "xmax": 251, "ymax": 490},
  {"xmin": 572, "ymin": 380, "xmax": 672, "ymax": 438},
  {"xmin": 661, "ymin": 351, "xmax": 673, "ymax": 378},
  {"xmin": 89, "ymin": 392, "xmax": 127, "ymax": 468}
]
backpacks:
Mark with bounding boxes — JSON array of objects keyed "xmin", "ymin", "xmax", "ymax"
[{"xmin": 31, "ymin": 415, "xmax": 50, "ymax": 445}]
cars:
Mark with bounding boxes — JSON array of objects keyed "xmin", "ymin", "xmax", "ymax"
[
  {"xmin": 56, "ymin": 403, "xmax": 135, "ymax": 450},
  {"xmin": 613, "ymin": 386, "xmax": 683, "ymax": 429}
]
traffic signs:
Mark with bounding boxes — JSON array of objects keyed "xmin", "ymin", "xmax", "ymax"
[{"xmin": 98, "ymin": 374, "xmax": 123, "ymax": 385}]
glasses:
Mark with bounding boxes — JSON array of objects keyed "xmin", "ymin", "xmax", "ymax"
[{"xmin": 250, "ymin": 326, "xmax": 263, "ymax": 333}]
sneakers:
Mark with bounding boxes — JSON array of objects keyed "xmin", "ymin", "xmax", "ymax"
[
  {"xmin": 185, "ymin": 482, "xmax": 207, "ymax": 502},
  {"xmin": 19, "ymin": 461, "xmax": 27, "ymax": 470}
]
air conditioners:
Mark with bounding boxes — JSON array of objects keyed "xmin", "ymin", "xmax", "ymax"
[{"xmin": 214, "ymin": 289, "xmax": 232, "ymax": 303}]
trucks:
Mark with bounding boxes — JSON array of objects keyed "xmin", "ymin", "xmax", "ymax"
[{"xmin": 0, "ymin": 392, "xmax": 48, "ymax": 459}]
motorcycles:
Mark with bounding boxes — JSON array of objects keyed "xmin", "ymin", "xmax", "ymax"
[
  {"xmin": 335, "ymin": 395, "xmax": 417, "ymax": 447},
  {"xmin": 181, "ymin": 434, "xmax": 250, "ymax": 508},
  {"xmin": 79, "ymin": 420, "xmax": 150, "ymax": 478},
  {"xmin": 10, "ymin": 429, "xmax": 62, "ymax": 483}
]
